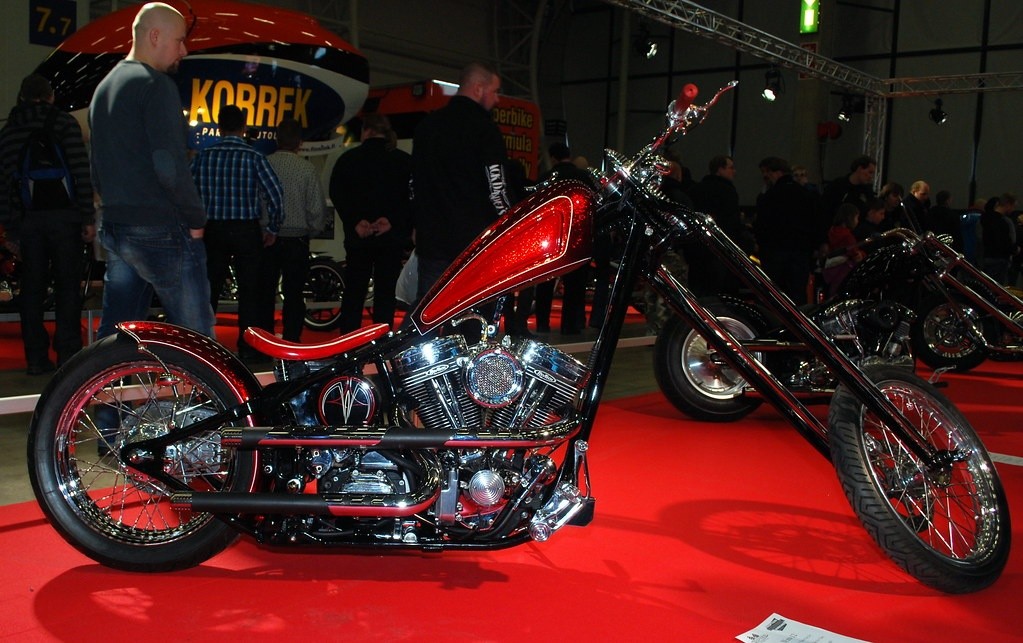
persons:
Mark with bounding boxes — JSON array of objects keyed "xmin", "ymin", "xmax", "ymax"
[
  {"xmin": 0, "ymin": 0, "xmax": 628, "ymax": 455},
  {"xmin": 632, "ymin": 148, "xmax": 1023, "ymax": 335}
]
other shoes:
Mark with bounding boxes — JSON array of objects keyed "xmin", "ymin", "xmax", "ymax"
[
  {"xmin": 239, "ymin": 348, "xmax": 277, "ymax": 369},
  {"xmin": 97, "ymin": 446, "xmax": 116, "ymax": 457},
  {"xmin": 32, "ymin": 363, "xmax": 57, "ymax": 376}
]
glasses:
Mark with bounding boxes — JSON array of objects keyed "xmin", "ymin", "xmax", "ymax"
[{"xmin": 793, "ymin": 172, "xmax": 807, "ymax": 177}]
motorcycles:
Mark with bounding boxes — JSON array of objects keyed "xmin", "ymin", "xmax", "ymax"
[
  {"xmin": 652, "ymin": 189, "xmax": 1023, "ymax": 421},
  {"xmin": 23, "ymin": 77, "xmax": 1014, "ymax": 597}
]
